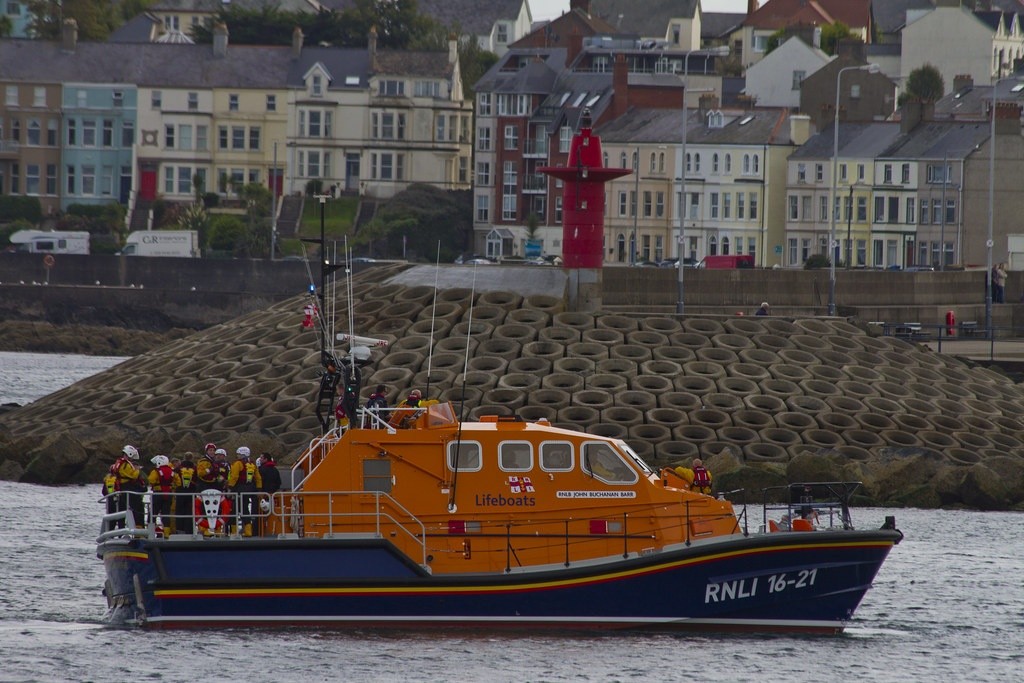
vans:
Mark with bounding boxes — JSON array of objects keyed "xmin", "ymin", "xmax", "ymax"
[{"xmin": 697, "ymin": 255, "xmax": 754, "ymax": 269}]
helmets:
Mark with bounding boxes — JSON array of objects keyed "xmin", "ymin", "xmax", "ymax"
[
  {"xmin": 156, "ymin": 456, "xmax": 169, "ymax": 468},
  {"xmin": 122, "ymin": 445, "xmax": 137, "ymax": 458},
  {"xmin": 205, "ymin": 444, "xmax": 216, "ymax": 451},
  {"xmin": 256, "ymin": 457, "xmax": 262, "ymax": 467},
  {"xmin": 236, "ymin": 446, "xmax": 250, "ymax": 456},
  {"xmin": 215, "ymin": 449, "xmax": 226, "ymax": 456}
]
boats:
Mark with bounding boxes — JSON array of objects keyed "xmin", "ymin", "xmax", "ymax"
[{"xmin": 94, "ymin": 143, "xmax": 905, "ymax": 641}]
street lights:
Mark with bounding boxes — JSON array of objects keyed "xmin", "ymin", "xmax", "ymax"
[
  {"xmin": 828, "ymin": 63, "xmax": 883, "ymax": 317},
  {"xmin": 675, "ymin": 45, "xmax": 734, "ymax": 312},
  {"xmin": 985, "ymin": 75, "xmax": 1024, "ymax": 342}
]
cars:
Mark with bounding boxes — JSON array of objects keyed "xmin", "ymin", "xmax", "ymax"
[
  {"xmin": 524, "ymin": 259, "xmax": 553, "ymax": 266},
  {"xmin": 629, "ymin": 257, "xmax": 700, "ymax": 269},
  {"xmin": 463, "ymin": 258, "xmax": 493, "ymax": 265}
]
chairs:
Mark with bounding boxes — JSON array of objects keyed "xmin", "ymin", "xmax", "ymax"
[
  {"xmin": 769, "ymin": 520, "xmax": 780, "ymax": 532},
  {"xmin": 794, "ymin": 520, "xmax": 812, "ymax": 532}
]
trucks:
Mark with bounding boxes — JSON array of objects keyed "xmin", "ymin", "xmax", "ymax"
[
  {"xmin": 114, "ymin": 229, "xmax": 201, "ymax": 259},
  {"xmin": 8, "ymin": 229, "xmax": 91, "ymax": 254}
]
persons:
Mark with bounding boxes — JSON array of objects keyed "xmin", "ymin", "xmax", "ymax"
[
  {"xmin": 148, "ymin": 455, "xmax": 181, "ymax": 539},
  {"xmin": 170, "ymin": 452, "xmax": 197, "ymax": 533},
  {"xmin": 588, "ymin": 447, "xmax": 624, "ymax": 480},
  {"xmin": 756, "ymin": 303, "xmax": 769, "ymax": 315},
  {"xmin": 251, "ymin": 452, "xmax": 281, "ymax": 536},
  {"xmin": 392, "ymin": 390, "xmax": 422, "ymax": 428},
  {"xmin": 675, "ymin": 459, "xmax": 712, "ymax": 494},
  {"xmin": 367, "ymin": 385, "xmax": 392, "ymax": 429},
  {"xmin": 196, "ymin": 443, "xmax": 231, "ymax": 537},
  {"xmin": 985, "ymin": 262, "xmax": 1008, "ymax": 303},
  {"xmin": 224, "ymin": 447, "xmax": 262, "ymax": 537},
  {"xmin": 102, "ymin": 445, "xmax": 146, "ymax": 539}
]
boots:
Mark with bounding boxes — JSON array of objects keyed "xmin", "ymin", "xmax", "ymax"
[
  {"xmin": 198, "ymin": 525, "xmax": 213, "ymax": 536},
  {"xmin": 242, "ymin": 524, "xmax": 252, "ymax": 536},
  {"xmin": 231, "ymin": 525, "xmax": 237, "ymax": 534},
  {"xmin": 163, "ymin": 527, "xmax": 170, "ymax": 539},
  {"xmin": 214, "ymin": 526, "xmax": 221, "ymax": 533}
]
